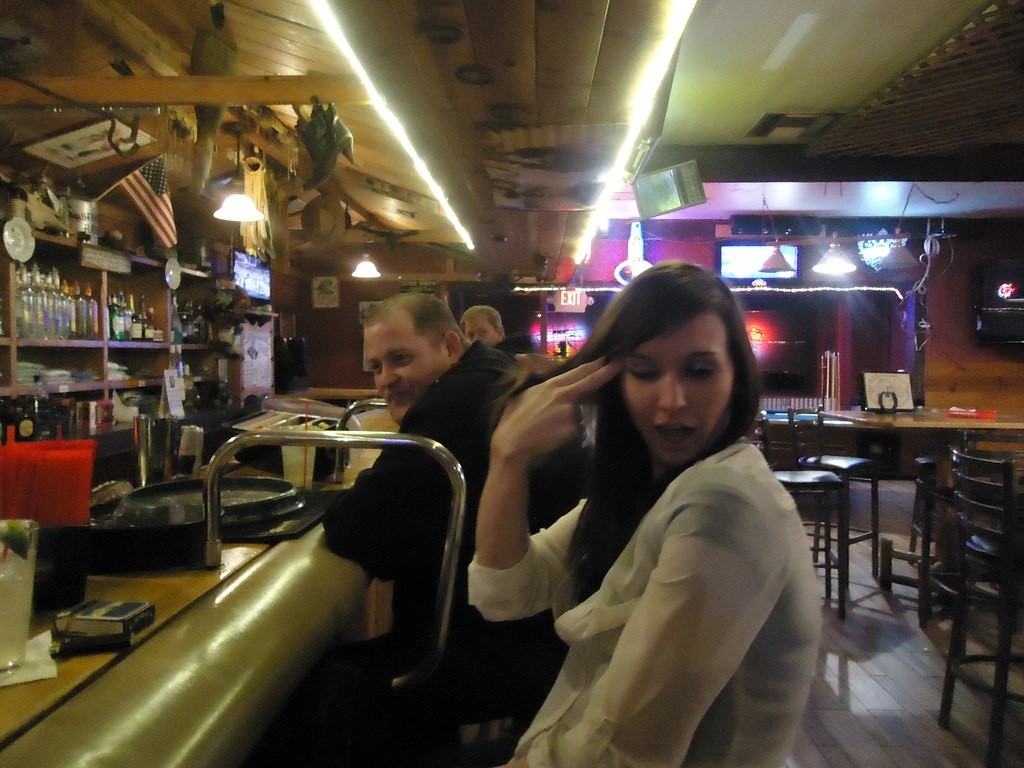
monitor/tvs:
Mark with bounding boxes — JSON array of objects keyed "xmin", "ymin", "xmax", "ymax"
[
  {"xmin": 975, "ymin": 257, "xmax": 1024, "ymax": 344},
  {"xmin": 717, "ymin": 241, "xmax": 802, "ymax": 286},
  {"xmin": 228, "ymin": 247, "xmax": 272, "ymax": 303}
]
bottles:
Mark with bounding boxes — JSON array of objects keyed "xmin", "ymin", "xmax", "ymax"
[
  {"xmin": 108, "ymin": 290, "xmax": 163, "ymax": 342},
  {"xmin": 15, "ymin": 263, "xmax": 99, "ymax": 340},
  {"xmin": 171, "ymin": 296, "xmax": 212, "ymax": 346}
]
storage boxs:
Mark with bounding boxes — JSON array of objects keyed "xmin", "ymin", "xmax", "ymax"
[{"xmin": 53, "ymin": 596, "xmax": 158, "ymax": 636}]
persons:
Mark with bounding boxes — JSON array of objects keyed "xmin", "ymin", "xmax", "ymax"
[
  {"xmin": 460, "ymin": 305, "xmax": 533, "ymax": 354},
  {"xmin": 468, "ymin": 262, "xmax": 821, "ymax": 768},
  {"xmin": 312, "ymin": 291, "xmax": 596, "ymax": 768}
]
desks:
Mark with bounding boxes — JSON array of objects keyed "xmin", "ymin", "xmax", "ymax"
[{"xmin": 764, "ymin": 408, "xmax": 1024, "ymax": 535}]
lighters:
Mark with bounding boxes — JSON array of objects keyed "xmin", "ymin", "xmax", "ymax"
[{"xmin": 48, "ymin": 632, "xmax": 132, "ymax": 658}]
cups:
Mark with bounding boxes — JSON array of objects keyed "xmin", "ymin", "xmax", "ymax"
[{"xmin": 0, "ymin": 518, "xmax": 38, "ymax": 672}]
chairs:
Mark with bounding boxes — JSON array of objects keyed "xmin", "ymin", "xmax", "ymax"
[
  {"xmin": 787, "ymin": 406, "xmax": 882, "ymax": 590},
  {"xmin": 759, "ymin": 417, "xmax": 851, "ymax": 621},
  {"xmin": 907, "ymin": 437, "xmax": 1024, "ymax": 767}
]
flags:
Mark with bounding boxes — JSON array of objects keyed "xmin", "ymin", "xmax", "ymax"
[{"xmin": 119, "ymin": 153, "xmax": 177, "ymax": 251}]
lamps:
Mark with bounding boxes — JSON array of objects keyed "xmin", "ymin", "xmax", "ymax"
[
  {"xmin": 879, "ymin": 227, "xmax": 918, "ymax": 269},
  {"xmin": 758, "ymin": 233, "xmax": 798, "ymax": 277},
  {"xmin": 811, "ymin": 229, "xmax": 856, "ymax": 275},
  {"xmin": 210, "ymin": 116, "xmax": 270, "ymax": 229},
  {"xmin": 348, "ymin": 241, "xmax": 383, "ymax": 279}
]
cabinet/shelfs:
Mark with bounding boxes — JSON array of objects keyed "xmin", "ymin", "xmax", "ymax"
[{"xmin": 0, "ymin": 216, "xmax": 218, "ymax": 404}]
